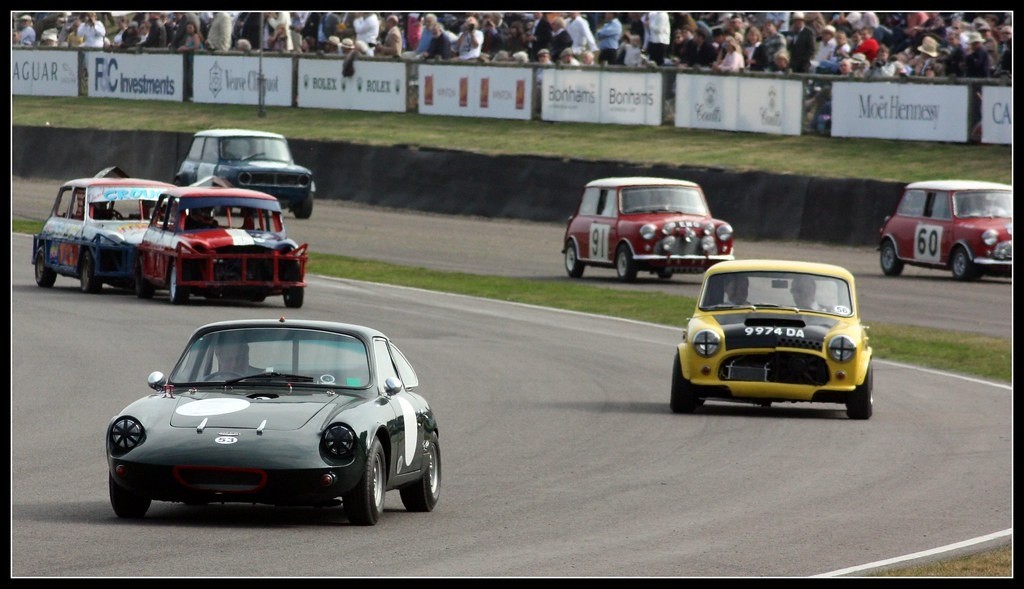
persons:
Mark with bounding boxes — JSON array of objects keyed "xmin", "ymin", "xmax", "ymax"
[
  {"xmin": 185, "ymin": 207, "xmax": 218, "ymax": 230},
  {"xmin": 723, "ymin": 275, "xmax": 751, "ymax": 305},
  {"xmin": 93, "ymin": 201, "xmax": 119, "ymax": 220},
  {"xmin": 215, "ymin": 342, "xmax": 266, "ymax": 379},
  {"xmin": 790, "ymin": 276, "xmax": 829, "ymax": 311},
  {"xmin": 12, "ymin": 12, "xmax": 1012, "ymax": 117}
]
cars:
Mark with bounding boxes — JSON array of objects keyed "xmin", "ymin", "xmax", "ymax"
[
  {"xmin": 878, "ymin": 179, "xmax": 1014, "ymax": 283},
  {"xmin": 562, "ymin": 177, "xmax": 736, "ymax": 282},
  {"xmin": 141, "ymin": 185, "xmax": 312, "ymax": 307},
  {"xmin": 670, "ymin": 261, "xmax": 875, "ymax": 419},
  {"xmin": 31, "ymin": 177, "xmax": 177, "ymax": 294},
  {"xmin": 175, "ymin": 129, "xmax": 316, "ymax": 218}
]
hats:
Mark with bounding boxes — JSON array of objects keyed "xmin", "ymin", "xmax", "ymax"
[
  {"xmin": 849, "ymin": 52, "xmax": 867, "ymax": 65},
  {"xmin": 339, "ymin": 37, "xmax": 356, "ymax": 49},
  {"xmin": 977, "ymin": 26, "xmax": 990, "ymax": 31},
  {"xmin": 45, "ymin": 33, "xmax": 58, "ymax": 42},
  {"xmin": 16, "ymin": 14, "xmax": 32, "ymax": 23},
  {"xmin": 823, "ymin": 25, "xmax": 837, "ymax": 37},
  {"xmin": 917, "ymin": 36, "xmax": 939, "ymax": 59},
  {"xmin": 559, "ymin": 47, "xmax": 572, "ymax": 58},
  {"xmin": 966, "ymin": 34, "xmax": 986, "ymax": 45},
  {"xmin": 324, "ymin": 36, "xmax": 340, "ymax": 47},
  {"xmin": 790, "ymin": 12, "xmax": 807, "ymax": 21},
  {"xmin": 537, "ymin": 48, "xmax": 549, "ymax": 56}
]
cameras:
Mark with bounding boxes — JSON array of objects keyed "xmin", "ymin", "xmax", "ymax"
[
  {"xmin": 468, "ymin": 23, "xmax": 475, "ymax": 29},
  {"xmin": 875, "ymin": 58, "xmax": 885, "ymax": 66}
]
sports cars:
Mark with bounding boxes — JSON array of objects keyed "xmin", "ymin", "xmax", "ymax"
[{"xmin": 104, "ymin": 320, "xmax": 442, "ymax": 527}]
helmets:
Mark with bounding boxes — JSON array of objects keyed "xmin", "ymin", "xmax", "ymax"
[{"xmin": 191, "ymin": 207, "xmax": 216, "ymax": 225}]
